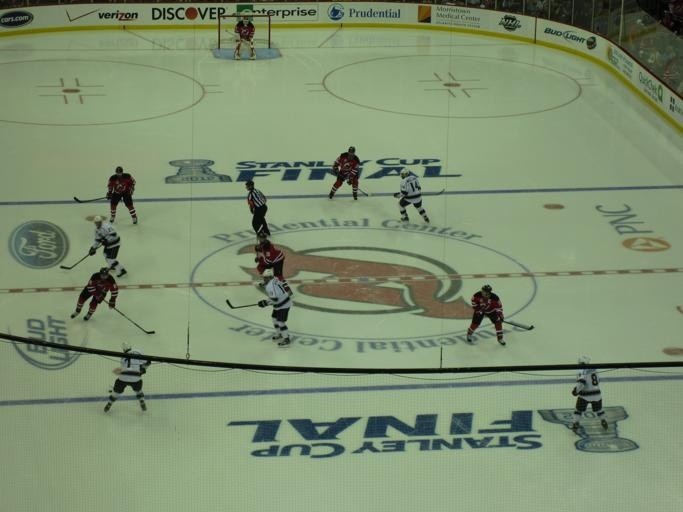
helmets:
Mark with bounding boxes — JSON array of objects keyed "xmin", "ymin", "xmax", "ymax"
[
  {"xmin": 256, "ymin": 244, "xmax": 260, "ymax": 251},
  {"xmin": 100, "ymin": 267, "xmax": 108, "ymax": 277},
  {"xmin": 122, "ymin": 342, "xmax": 131, "ymax": 351},
  {"xmin": 115, "ymin": 166, "xmax": 124, "ymax": 174},
  {"xmin": 482, "ymin": 286, "xmax": 493, "ymax": 291},
  {"xmin": 245, "ymin": 180, "xmax": 254, "ymax": 187},
  {"xmin": 94, "ymin": 216, "xmax": 102, "ymax": 222},
  {"xmin": 348, "ymin": 147, "xmax": 356, "ymax": 152},
  {"xmin": 578, "ymin": 355, "xmax": 590, "ymax": 365},
  {"xmin": 400, "ymin": 168, "xmax": 408, "ymax": 176},
  {"xmin": 264, "ymin": 270, "xmax": 274, "ymax": 277}
]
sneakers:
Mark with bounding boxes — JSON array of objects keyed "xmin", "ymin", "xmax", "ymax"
[
  {"xmin": 71, "ymin": 311, "xmax": 91, "ymax": 320},
  {"xmin": 355, "ymin": 198, "xmax": 358, "ymax": 201},
  {"xmin": 132, "ymin": 217, "xmax": 136, "ymax": 224},
  {"xmin": 104, "ymin": 406, "xmax": 110, "ymax": 413},
  {"xmin": 272, "ymin": 335, "xmax": 291, "ymax": 345},
  {"xmin": 108, "ymin": 216, "xmax": 114, "ymax": 222},
  {"xmin": 602, "ymin": 419, "xmax": 608, "ymax": 429},
  {"xmin": 329, "ymin": 195, "xmax": 332, "ymax": 199},
  {"xmin": 401, "ymin": 218, "xmax": 409, "ymax": 222},
  {"xmin": 426, "ymin": 220, "xmax": 429, "ymax": 223},
  {"xmin": 116, "ymin": 269, "xmax": 125, "ymax": 277},
  {"xmin": 468, "ymin": 335, "xmax": 473, "ymax": 341},
  {"xmin": 142, "ymin": 404, "xmax": 146, "ymax": 411},
  {"xmin": 575, "ymin": 421, "xmax": 580, "ymax": 429},
  {"xmin": 498, "ymin": 341, "xmax": 507, "ymax": 345}
]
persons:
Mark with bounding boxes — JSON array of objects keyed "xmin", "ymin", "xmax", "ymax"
[
  {"xmin": 610, "ymin": 1, "xmax": 683, "ymax": 98},
  {"xmin": 69, "ymin": 267, "xmax": 118, "ymax": 320},
  {"xmin": 251, "ymin": 230, "xmax": 293, "ymax": 296},
  {"xmin": 326, "ymin": 145, "xmax": 360, "ymax": 200},
  {"xmin": 101, "ymin": 341, "xmax": 151, "ymax": 413},
  {"xmin": 227, "ymin": 17, "xmax": 256, "ymax": 60},
  {"xmin": 392, "ymin": 168, "xmax": 430, "ymax": 224},
  {"xmin": 464, "ymin": 284, "xmax": 506, "ymax": 346},
  {"xmin": 104, "ymin": 166, "xmax": 138, "ymax": 226},
  {"xmin": 256, "ymin": 269, "xmax": 293, "ymax": 346},
  {"xmin": 243, "ymin": 180, "xmax": 271, "ymax": 237},
  {"xmin": 427, "ymin": 0, "xmax": 614, "ymax": 41},
  {"xmin": 564, "ymin": 354, "xmax": 608, "ymax": 430},
  {"xmin": 87, "ymin": 215, "xmax": 127, "ymax": 277}
]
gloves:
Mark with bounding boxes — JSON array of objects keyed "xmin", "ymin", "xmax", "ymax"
[
  {"xmin": 89, "ymin": 248, "xmax": 96, "ymax": 255},
  {"xmin": 394, "ymin": 193, "xmax": 400, "ymax": 198},
  {"xmin": 108, "ymin": 299, "xmax": 116, "ymax": 308},
  {"xmin": 347, "ymin": 178, "xmax": 353, "ymax": 185},
  {"xmin": 105, "ymin": 192, "xmax": 112, "ymax": 200},
  {"xmin": 258, "ymin": 300, "xmax": 268, "ymax": 308}
]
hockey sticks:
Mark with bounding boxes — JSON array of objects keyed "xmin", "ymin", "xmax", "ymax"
[
  {"xmin": 102, "ymin": 299, "xmax": 155, "ymax": 334},
  {"xmin": 339, "ymin": 172, "xmax": 375, "ymax": 196},
  {"xmin": 421, "ymin": 189, "xmax": 445, "ymax": 195},
  {"xmin": 60, "ymin": 245, "xmax": 102, "ymax": 269},
  {"xmin": 225, "ymin": 29, "xmax": 256, "ymax": 47},
  {"xmin": 502, "ymin": 319, "xmax": 533, "ymax": 330},
  {"xmin": 226, "ymin": 299, "xmax": 258, "ymax": 308},
  {"xmin": 73, "ymin": 196, "xmax": 107, "ymax": 202}
]
